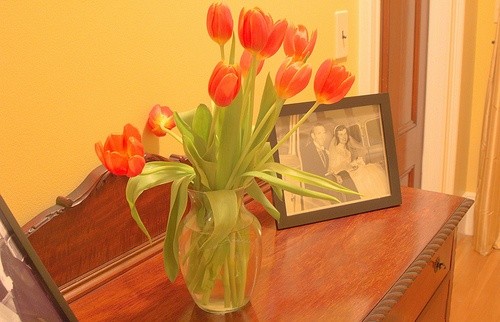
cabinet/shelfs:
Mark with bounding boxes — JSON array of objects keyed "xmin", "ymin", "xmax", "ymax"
[{"xmin": 0, "ymin": 152, "xmax": 477, "ymax": 321}]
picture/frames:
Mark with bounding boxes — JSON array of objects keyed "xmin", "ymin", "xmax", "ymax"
[{"xmin": 267, "ymin": 92, "xmax": 404, "ymax": 231}]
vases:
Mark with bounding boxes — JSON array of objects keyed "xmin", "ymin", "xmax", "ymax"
[{"xmin": 93, "ymin": 3, "xmax": 351, "ymax": 316}]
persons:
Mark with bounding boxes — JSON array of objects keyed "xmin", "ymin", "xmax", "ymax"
[
  {"xmin": 302, "ymin": 124, "xmax": 362, "ymax": 204},
  {"xmin": 328, "ymin": 124, "xmax": 391, "ymax": 199}
]
